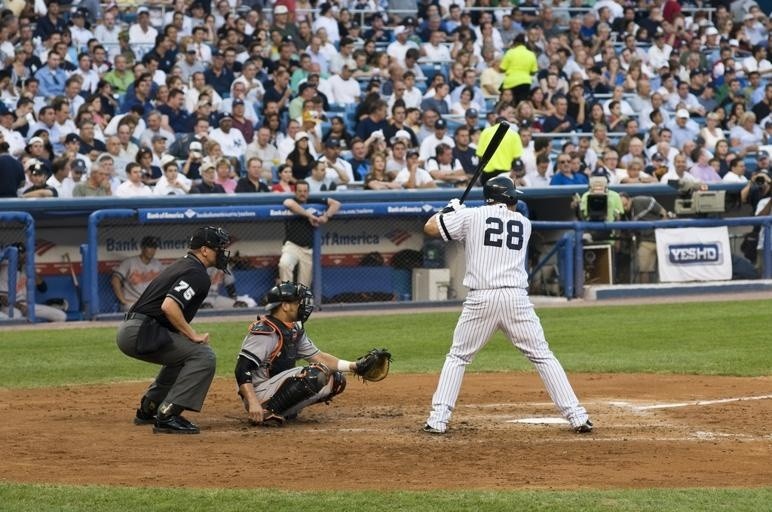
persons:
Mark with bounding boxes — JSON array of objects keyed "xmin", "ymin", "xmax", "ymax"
[
  {"xmin": 2, "ymin": 0, "xmax": 772, "ymax": 200},
  {"xmin": 2, "ymin": 242, "xmax": 68, "ymax": 323},
  {"xmin": 422, "ymin": 177, "xmax": 593, "ymax": 434},
  {"xmin": 579, "ymin": 166, "xmax": 770, "ymax": 285},
  {"xmin": 116, "ymin": 225, "xmax": 231, "ymax": 434},
  {"xmin": 278, "ymin": 180, "xmax": 343, "ymax": 291},
  {"xmin": 235, "ymin": 282, "xmax": 366, "ymax": 428},
  {"xmin": 111, "ymin": 234, "xmax": 254, "ymax": 311}
]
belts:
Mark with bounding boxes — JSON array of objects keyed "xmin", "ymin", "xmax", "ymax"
[{"xmin": 124, "ymin": 313, "xmax": 148, "ymax": 321}]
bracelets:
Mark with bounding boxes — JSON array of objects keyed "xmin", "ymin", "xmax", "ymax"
[{"xmin": 338, "ymin": 360, "xmax": 351, "ymax": 372}]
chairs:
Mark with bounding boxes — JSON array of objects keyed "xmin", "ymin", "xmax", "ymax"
[{"xmin": 238, "ymin": 28, "xmax": 772, "ymax": 180}]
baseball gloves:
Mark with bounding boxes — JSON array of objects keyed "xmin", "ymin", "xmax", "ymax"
[{"xmin": 354, "ymin": 347, "xmax": 391, "ymax": 381}]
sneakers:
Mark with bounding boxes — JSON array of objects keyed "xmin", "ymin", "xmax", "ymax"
[
  {"xmin": 574, "ymin": 419, "xmax": 593, "ymax": 434},
  {"xmin": 423, "ymin": 423, "xmax": 442, "ymax": 433},
  {"xmin": 134, "ymin": 409, "xmax": 199, "ymax": 434}
]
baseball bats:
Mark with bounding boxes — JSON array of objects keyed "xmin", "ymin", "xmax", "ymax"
[
  {"xmin": 460, "ymin": 121, "xmax": 510, "ymax": 204},
  {"xmin": 65, "ymin": 252, "xmax": 81, "ymax": 303}
]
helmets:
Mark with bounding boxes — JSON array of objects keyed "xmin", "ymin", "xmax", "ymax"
[
  {"xmin": 265, "ymin": 284, "xmax": 314, "ymax": 323},
  {"xmin": 189, "ymin": 226, "xmax": 231, "ymax": 275},
  {"xmin": 483, "ymin": 176, "xmax": 523, "ymax": 205}
]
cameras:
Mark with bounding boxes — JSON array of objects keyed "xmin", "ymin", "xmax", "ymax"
[{"xmin": 755, "ymin": 176, "xmax": 765, "ymax": 186}]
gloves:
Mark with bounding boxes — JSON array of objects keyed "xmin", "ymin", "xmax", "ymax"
[{"xmin": 441, "ymin": 198, "xmax": 466, "ymax": 212}]
